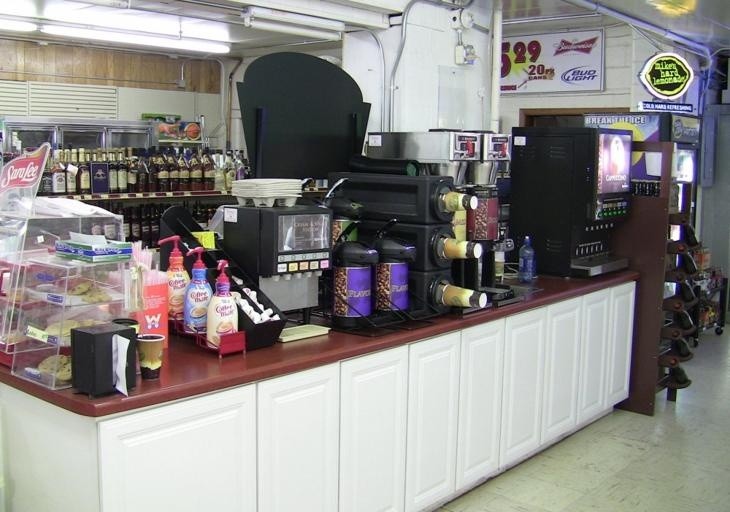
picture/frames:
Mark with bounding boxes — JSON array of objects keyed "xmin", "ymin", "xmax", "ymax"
[{"xmin": 501, "ymin": 27, "xmax": 605, "ymax": 96}]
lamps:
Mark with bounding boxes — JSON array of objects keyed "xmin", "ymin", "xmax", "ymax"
[{"xmin": 241, "ymin": 7, "xmax": 344, "ymax": 42}]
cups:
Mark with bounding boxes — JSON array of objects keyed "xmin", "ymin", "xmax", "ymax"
[{"xmin": 110, "ymin": 315, "xmax": 165, "ymax": 381}]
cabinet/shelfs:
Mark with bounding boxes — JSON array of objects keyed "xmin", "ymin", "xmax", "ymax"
[
  {"xmin": 12, "ymin": 258, "xmax": 124, "ymax": 390},
  {"xmin": 1, "ymin": 116, "xmax": 160, "ymax": 152}
]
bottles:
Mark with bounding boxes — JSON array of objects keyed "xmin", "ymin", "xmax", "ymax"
[
  {"xmin": 517, "ymin": 236, "xmax": 536, "ymax": 283},
  {"xmin": 2, "ymin": 140, "xmax": 247, "ymax": 194},
  {"xmin": 82, "ymin": 201, "xmax": 240, "ymax": 245},
  {"xmin": 426, "ymin": 181, "xmax": 487, "ymax": 314}
]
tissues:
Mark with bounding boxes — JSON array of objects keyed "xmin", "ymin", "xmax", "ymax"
[{"xmin": 71, "ymin": 322, "xmax": 137, "ymax": 396}]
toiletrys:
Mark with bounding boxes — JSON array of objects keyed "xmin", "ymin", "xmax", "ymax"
[{"xmin": 157, "ymin": 235, "xmax": 239, "ymax": 350}]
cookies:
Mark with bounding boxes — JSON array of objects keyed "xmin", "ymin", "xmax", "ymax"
[{"xmin": 9, "ymin": 279, "xmax": 112, "ymax": 380}]
